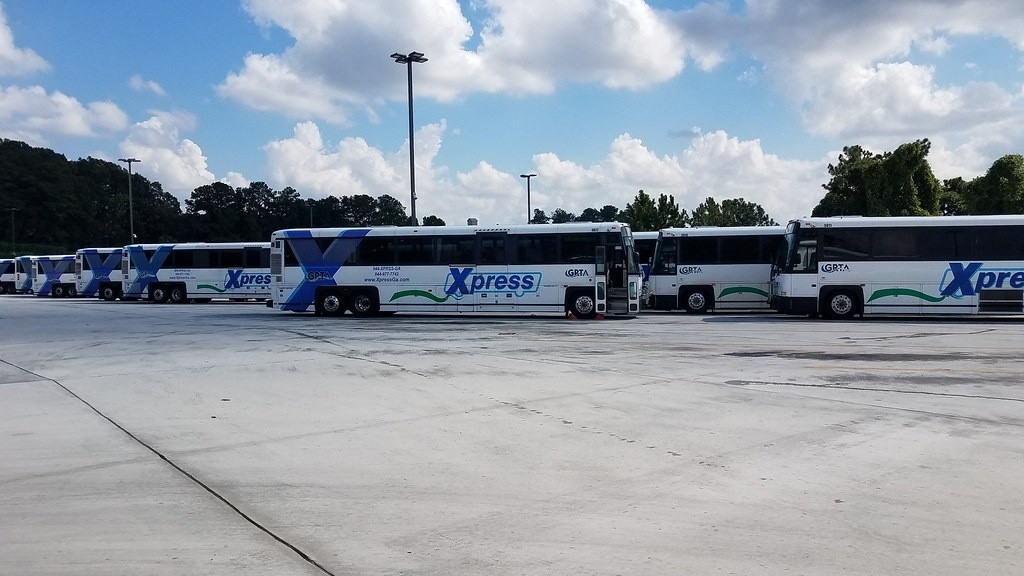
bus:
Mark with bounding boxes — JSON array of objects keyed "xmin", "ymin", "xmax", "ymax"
[
  {"xmin": 766, "ymin": 215, "xmax": 1024, "ymax": 320},
  {"xmin": 0, "ymin": 259, "xmax": 15, "ymax": 294},
  {"xmin": 648, "ymin": 226, "xmax": 817, "ymax": 314},
  {"xmin": 75, "ymin": 248, "xmax": 123, "ymax": 300},
  {"xmin": 31, "ymin": 255, "xmax": 76, "ymax": 297},
  {"xmin": 15, "ymin": 256, "xmax": 33, "ymax": 289},
  {"xmin": 595, "ymin": 232, "xmax": 676, "ymax": 297},
  {"xmin": 270, "ymin": 221, "xmax": 643, "ymax": 320},
  {"xmin": 121, "ymin": 242, "xmax": 271, "ymax": 304}
]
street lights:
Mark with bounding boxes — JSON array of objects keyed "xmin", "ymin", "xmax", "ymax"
[
  {"xmin": 118, "ymin": 158, "xmax": 141, "ymax": 244},
  {"xmin": 390, "ymin": 51, "xmax": 428, "ymax": 227},
  {"xmin": 9, "ymin": 208, "xmax": 21, "ymax": 259},
  {"xmin": 520, "ymin": 173, "xmax": 537, "ymax": 223}
]
